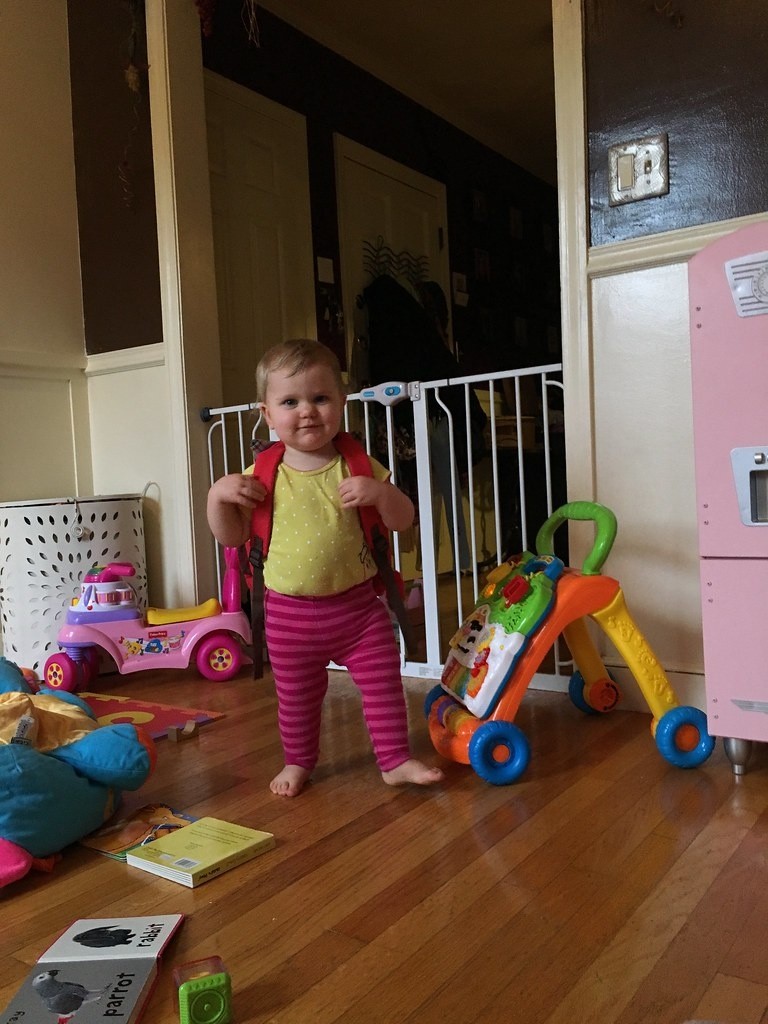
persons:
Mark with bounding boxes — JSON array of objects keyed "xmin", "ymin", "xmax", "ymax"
[{"xmin": 206, "ymin": 339, "xmax": 445, "ymax": 797}]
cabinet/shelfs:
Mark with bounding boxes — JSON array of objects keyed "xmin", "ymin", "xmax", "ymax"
[
  {"xmin": 498, "ymin": 438, "xmax": 567, "ymax": 559},
  {"xmin": 688, "ymin": 217, "xmax": 768, "ymax": 775}
]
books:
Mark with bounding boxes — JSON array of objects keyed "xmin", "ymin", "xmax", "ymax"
[
  {"xmin": 126, "ymin": 817, "xmax": 276, "ymax": 888},
  {"xmin": 0, "ymin": 913, "xmax": 185, "ymax": 1024}
]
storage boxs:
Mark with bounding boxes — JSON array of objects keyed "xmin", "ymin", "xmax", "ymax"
[{"xmin": 486, "ymin": 416, "xmax": 536, "ymax": 449}]
